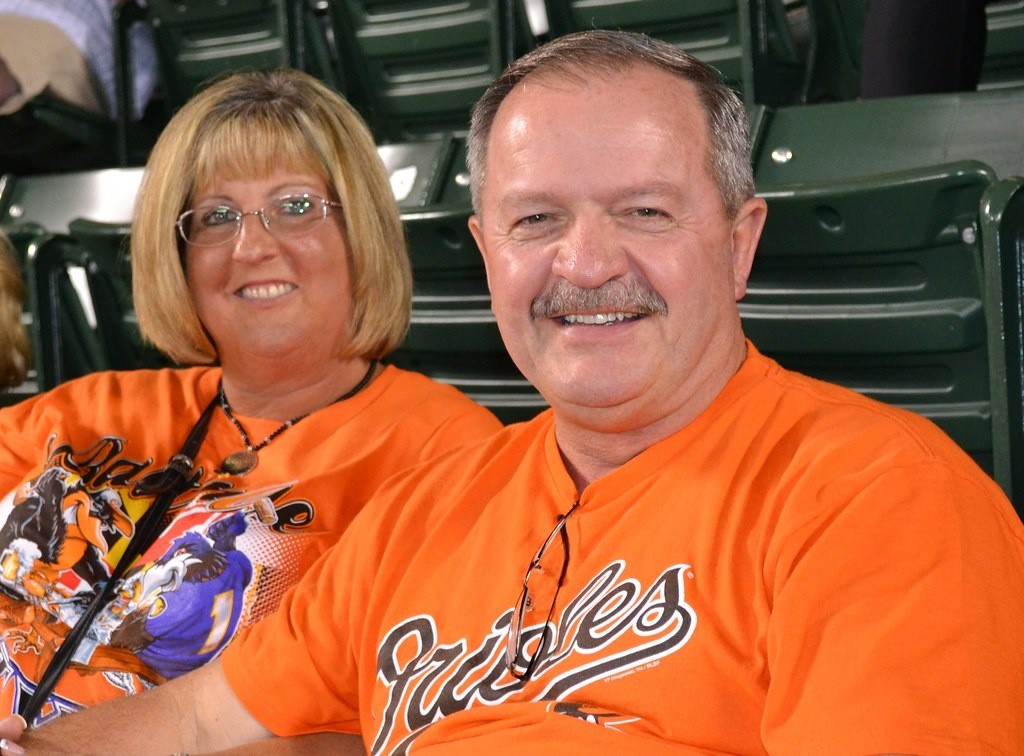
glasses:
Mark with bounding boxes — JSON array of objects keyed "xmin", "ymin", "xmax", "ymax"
[{"xmin": 175, "ymin": 194, "xmax": 342, "ymax": 247}]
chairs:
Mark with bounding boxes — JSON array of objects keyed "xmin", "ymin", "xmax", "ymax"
[{"xmin": 1, "ymin": 0, "xmax": 1024, "ymax": 520}]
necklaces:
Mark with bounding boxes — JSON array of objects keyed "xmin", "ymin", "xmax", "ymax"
[{"xmin": 218, "ymin": 359, "xmax": 377, "ymax": 476}]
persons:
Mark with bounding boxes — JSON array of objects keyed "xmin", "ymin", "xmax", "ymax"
[
  {"xmin": 0, "ymin": 68, "xmax": 508, "ymax": 756},
  {"xmin": 0, "ymin": 29, "xmax": 1024, "ymax": 756}
]
